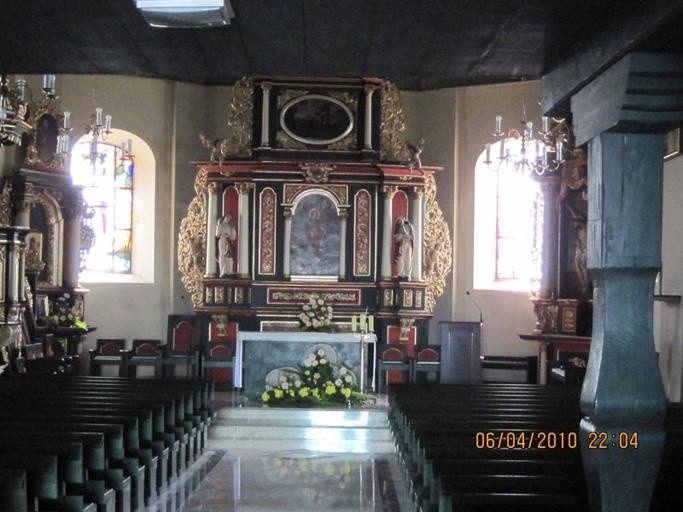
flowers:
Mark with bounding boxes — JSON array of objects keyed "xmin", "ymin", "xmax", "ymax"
[
  {"xmin": 259, "ymin": 348, "xmax": 368, "ymax": 407},
  {"xmin": 296, "ymin": 292, "xmax": 341, "ymax": 333},
  {"xmin": 39, "ymin": 292, "xmax": 89, "ymax": 333}
]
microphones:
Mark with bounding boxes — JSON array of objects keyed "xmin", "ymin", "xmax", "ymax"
[{"xmin": 466, "ymin": 291, "xmax": 482, "ymax": 322}]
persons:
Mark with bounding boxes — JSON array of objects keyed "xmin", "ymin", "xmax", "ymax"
[
  {"xmin": 303, "ymin": 208, "xmax": 323, "ymax": 252},
  {"xmin": 392, "ymin": 214, "xmax": 416, "ymax": 282},
  {"xmin": 214, "ymin": 211, "xmax": 236, "ymax": 278}
]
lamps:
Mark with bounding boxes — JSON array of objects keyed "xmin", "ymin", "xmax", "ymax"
[
  {"xmin": 481, "ymin": 76, "xmax": 567, "ymax": 176},
  {"xmin": 55, "ymin": 74, "xmax": 136, "ymax": 175}
]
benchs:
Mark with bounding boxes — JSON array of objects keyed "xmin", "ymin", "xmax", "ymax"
[
  {"xmin": 387, "ymin": 382, "xmax": 683, "ymax": 512},
  {"xmin": 0, "ymin": 372, "xmax": 219, "ymax": 512}
]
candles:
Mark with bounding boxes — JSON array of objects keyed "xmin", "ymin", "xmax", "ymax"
[
  {"xmin": 368, "ymin": 315, "xmax": 374, "ymax": 332},
  {"xmin": 43, "ymin": 74, "xmax": 51, "ymax": 88},
  {"xmin": 352, "ymin": 315, "xmax": 357, "ymax": 332},
  {"xmin": 360, "ymin": 314, "xmax": 365, "ymax": 330},
  {"xmin": 47, "ymin": 74, "xmax": 55, "ymax": 95}
]
